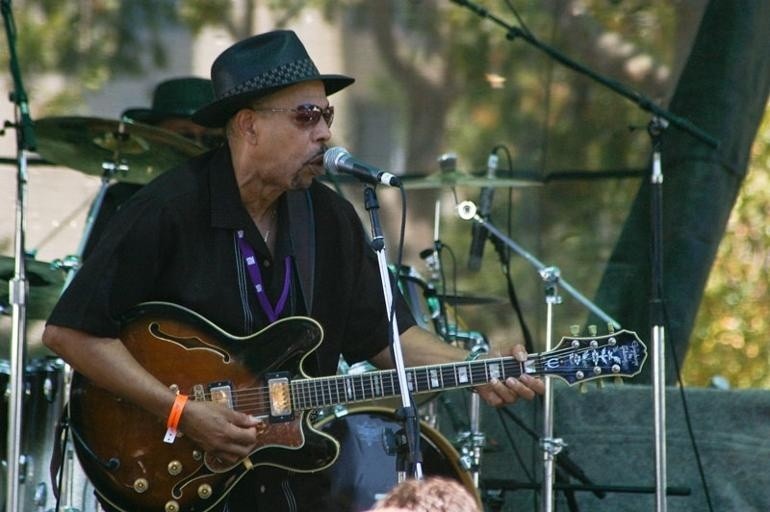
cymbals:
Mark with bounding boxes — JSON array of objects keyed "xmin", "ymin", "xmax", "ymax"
[
  {"xmin": 430, "ymin": 288, "xmax": 507, "ymax": 303},
  {"xmin": 23, "ymin": 116, "xmax": 209, "ymax": 185},
  {"xmin": 380, "ymin": 171, "xmax": 546, "ymax": 188},
  {"xmin": 1, "ymin": 256, "xmax": 85, "ymax": 318}
]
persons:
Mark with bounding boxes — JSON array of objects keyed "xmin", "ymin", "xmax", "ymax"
[
  {"xmin": 42, "ymin": 30, "xmax": 545, "ymax": 512},
  {"xmin": 77, "ymin": 76, "xmax": 226, "ymax": 264},
  {"xmin": 372, "ymin": 476, "xmax": 480, "ymax": 510}
]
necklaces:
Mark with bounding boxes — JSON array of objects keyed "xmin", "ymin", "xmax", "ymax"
[{"xmin": 264, "ymin": 204, "xmax": 277, "ymax": 243}]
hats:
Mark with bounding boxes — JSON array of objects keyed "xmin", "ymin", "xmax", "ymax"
[
  {"xmin": 191, "ymin": 30, "xmax": 355, "ymax": 128},
  {"xmin": 122, "ymin": 79, "xmax": 215, "ymax": 124}
]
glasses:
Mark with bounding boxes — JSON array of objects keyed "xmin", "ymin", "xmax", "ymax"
[{"xmin": 254, "ymin": 104, "xmax": 334, "ymax": 128}]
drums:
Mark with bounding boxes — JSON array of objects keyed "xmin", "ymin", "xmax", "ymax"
[{"xmin": 1, "ymin": 315, "xmax": 68, "ymax": 511}]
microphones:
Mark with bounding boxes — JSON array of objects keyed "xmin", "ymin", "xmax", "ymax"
[
  {"xmin": 323, "ymin": 147, "xmax": 402, "ymax": 188},
  {"xmin": 467, "ymin": 154, "xmax": 500, "ymax": 273}
]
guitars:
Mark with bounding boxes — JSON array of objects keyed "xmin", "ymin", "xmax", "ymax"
[
  {"xmin": 255, "ymin": 406, "xmax": 484, "ymax": 511},
  {"xmin": 69, "ymin": 301, "xmax": 647, "ymax": 510}
]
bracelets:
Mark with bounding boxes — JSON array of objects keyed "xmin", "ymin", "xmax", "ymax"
[
  {"xmin": 163, "ymin": 394, "xmax": 188, "ymax": 443},
  {"xmin": 464, "ymin": 351, "xmax": 488, "ymax": 393}
]
